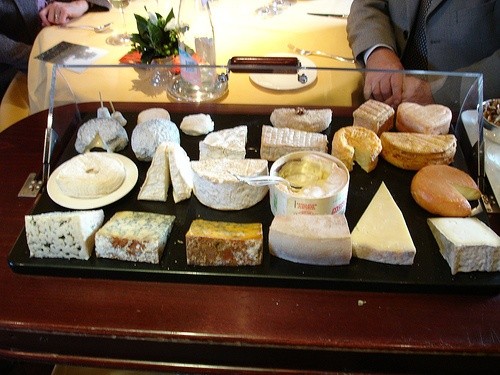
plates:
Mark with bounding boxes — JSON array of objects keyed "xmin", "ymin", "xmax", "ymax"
[
  {"xmin": 250, "ymin": 52, "xmax": 318, "ymax": 90},
  {"xmin": 47, "ymin": 154, "xmax": 138, "ymax": 211}
]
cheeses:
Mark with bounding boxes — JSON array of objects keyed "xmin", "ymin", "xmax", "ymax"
[{"xmin": 23, "ymin": 99, "xmax": 500, "ymax": 275}]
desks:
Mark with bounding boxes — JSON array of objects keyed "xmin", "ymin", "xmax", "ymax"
[
  {"xmin": 0, "ymin": 102, "xmax": 500, "ymax": 375},
  {"xmin": 26, "ymin": 0, "xmax": 366, "ymax": 114}
]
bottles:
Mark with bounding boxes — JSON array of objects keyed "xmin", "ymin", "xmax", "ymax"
[{"xmin": 177, "ymin": 1, "xmax": 214, "ymax": 89}]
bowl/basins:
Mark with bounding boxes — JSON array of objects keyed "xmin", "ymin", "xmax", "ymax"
[
  {"xmin": 268, "ymin": 150, "xmax": 350, "ymax": 218},
  {"xmin": 478, "ymin": 100, "xmax": 500, "ymax": 145}
]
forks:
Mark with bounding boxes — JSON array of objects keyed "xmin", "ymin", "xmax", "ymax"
[{"xmin": 287, "ymin": 45, "xmax": 355, "ymax": 63}]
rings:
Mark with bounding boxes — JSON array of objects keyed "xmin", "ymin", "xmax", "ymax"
[{"xmin": 54, "ymin": 15, "xmax": 59, "ymax": 19}]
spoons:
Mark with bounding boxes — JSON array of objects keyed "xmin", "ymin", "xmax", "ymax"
[{"xmin": 61, "ymin": 23, "xmax": 111, "ymax": 32}]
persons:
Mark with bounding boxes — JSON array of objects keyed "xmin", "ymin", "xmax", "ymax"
[
  {"xmin": 0, "ymin": 0, "xmax": 113, "ymax": 106},
  {"xmin": 347, "ymin": 0, "xmax": 500, "ymax": 113}
]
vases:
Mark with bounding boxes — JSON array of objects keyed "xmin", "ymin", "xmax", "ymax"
[{"xmin": 149, "ymin": 55, "xmax": 177, "ymax": 92}]
